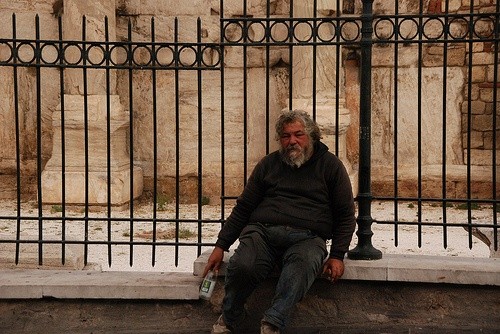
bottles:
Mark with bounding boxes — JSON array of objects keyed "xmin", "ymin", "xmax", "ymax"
[{"xmin": 199, "ymin": 264, "xmax": 217, "ymax": 300}]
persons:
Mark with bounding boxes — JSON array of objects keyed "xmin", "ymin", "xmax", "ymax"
[{"xmin": 203, "ymin": 110, "xmax": 356, "ymax": 334}]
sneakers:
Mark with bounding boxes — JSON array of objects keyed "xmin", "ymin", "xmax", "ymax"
[
  {"xmin": 261, "ymin": 319, "xmax": 280, "ymax": 334},
  {"xmin": 210, "ymin": 312, "xmax": 232, "ymax": 334}
]
